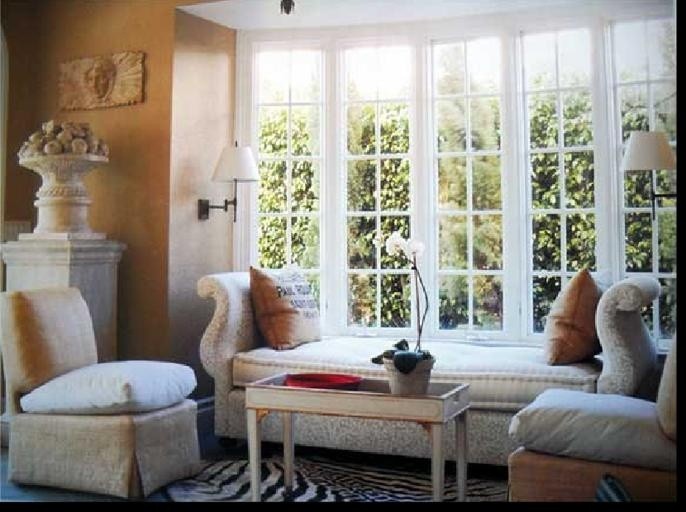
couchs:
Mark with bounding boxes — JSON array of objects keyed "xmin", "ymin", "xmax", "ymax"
[{"xmin": 196, "ymin": 269, "xmax": 662, "ymax": 467}]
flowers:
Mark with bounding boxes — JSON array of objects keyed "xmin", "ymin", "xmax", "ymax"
[{"xmin": 371, "ymin": 228, "xmax": 429, "ymax": 375}]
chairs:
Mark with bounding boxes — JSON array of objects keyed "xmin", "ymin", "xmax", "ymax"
[
  {"xmin": 0, "ymin": 286, "xmax": 202, "ymax": 500},
  {"xmin": 506, "ymin": 332, "xmax": 676, "ymax": 503}
]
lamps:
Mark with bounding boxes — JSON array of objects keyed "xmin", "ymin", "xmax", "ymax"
[
  {"xmin": 197, "ymin": 139, "xmax": 260, "ymax": 223},
  {"xmin": 619, "ymin": 131, "xmax": 676, "ymax": 220},
  {"xmin": 281, "ymin": 0, "xmax": 295, "ymax": 16}
]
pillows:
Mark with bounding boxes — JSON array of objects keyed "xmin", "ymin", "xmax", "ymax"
[
  {"xmin": 545, "ymin": 267, "xmax": 606, "ymax": 366},
  {"xmin": 508, "ymin": 387, "xmax": 675, "ymax": 472},
  {"xmin": 249, "ymin": 264, "xmax": 323, "ymax": 350},
  {"xmin": 20, "ymin": 359, "xmax": 197, "ymax": 418}
]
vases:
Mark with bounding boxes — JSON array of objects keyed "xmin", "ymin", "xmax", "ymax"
[{"xmin": 382, "ymin": 355, "xmax": 438, "ymax": 399}]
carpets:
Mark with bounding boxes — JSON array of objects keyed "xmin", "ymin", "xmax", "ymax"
[{"xmin": 164, "ymin": 456, "xmax": 509, "ymax": 501}]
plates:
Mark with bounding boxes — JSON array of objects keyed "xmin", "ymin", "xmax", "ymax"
[{"xmin": 286, "ymin": 373, "xmax": 363, "ymax": 388}]
